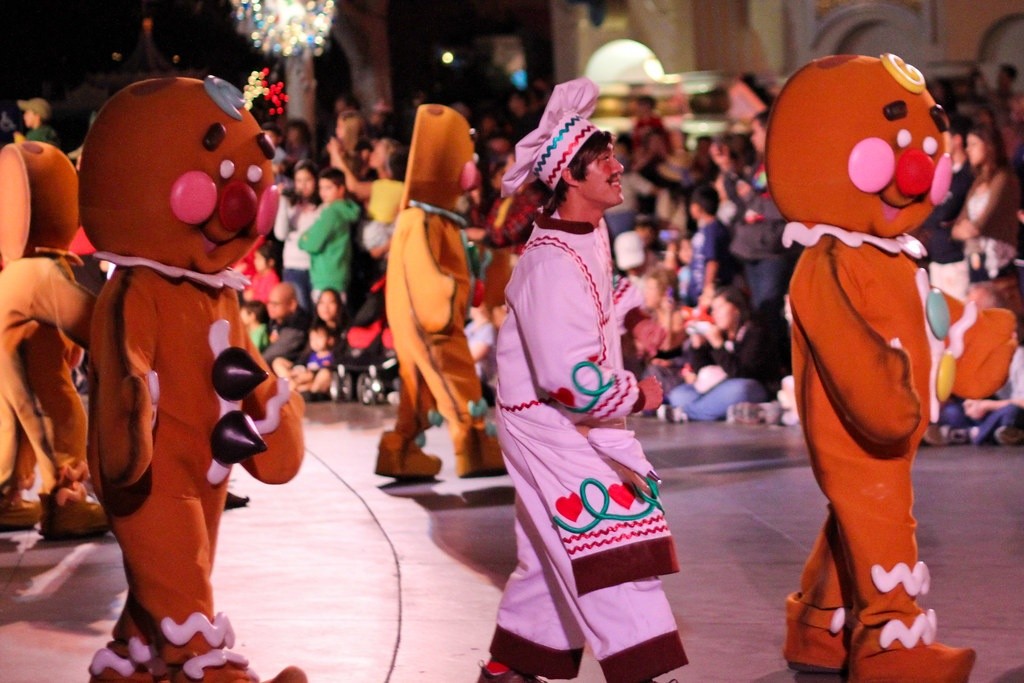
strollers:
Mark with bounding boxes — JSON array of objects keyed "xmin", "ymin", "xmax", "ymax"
[{"xmin": 327, "ymin": 269, "xmax": 398, "ymax": 405}]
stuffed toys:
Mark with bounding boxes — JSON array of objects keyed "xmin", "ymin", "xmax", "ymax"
[
  {"xmin": 372, "ymin": 103, "xmax": 507, "ymax": 482},
  {"xmin": 0, "ymin": 139, "xmax": 108, "ymax": 536},
  {"xmin": 77, "ymin": 76, "xmax": 309, "ymax": 682},
  {"xmin": 764, "ymin": 52, "xmax": 974, "ymax": 682}
]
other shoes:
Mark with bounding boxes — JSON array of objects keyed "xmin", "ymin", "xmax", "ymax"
[
  {"xmin": 993, "ymin": 424, "xmax": 1024, "ymax": 443},
  {"xmin": 656, "ymin": 403, "xmax": 687, "ymax": 423},
  {"xmin": 949, "ymin": 429, "xmax": 977, "ymax": 445},
  {"xmin": 923, "ymin": 426, "xmax": 951, "ymax": 445},
  {"xmin": 224, "ymin": 492, "xmax": 249, "ymax": 509}
]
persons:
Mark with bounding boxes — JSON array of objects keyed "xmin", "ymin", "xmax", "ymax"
[
  {"xmin": 238, "ymin": 93, "xmax": 412, "ymax": 407},
  {"xmin": 598, "ymin": 76, "xmax": 804, "ymax": 425},
  {"xmin": 903, "ymin": 62, "xmax": 1024, "ymax": 445},
  {"xmin": 0, "ymin": 96, "xmax": 109, "ymax": 394},
  {"xmin": 476, "ymin": 75, "xmax": 692, "ymax": 683},
  {"xmin": 463, "ymin": 77, "xmax": 551, "ymax": 406}
]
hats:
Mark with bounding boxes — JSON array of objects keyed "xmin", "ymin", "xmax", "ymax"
[{"xmin": 501, "ymin": 75, "xmax": 600, "ymax": 201}]
